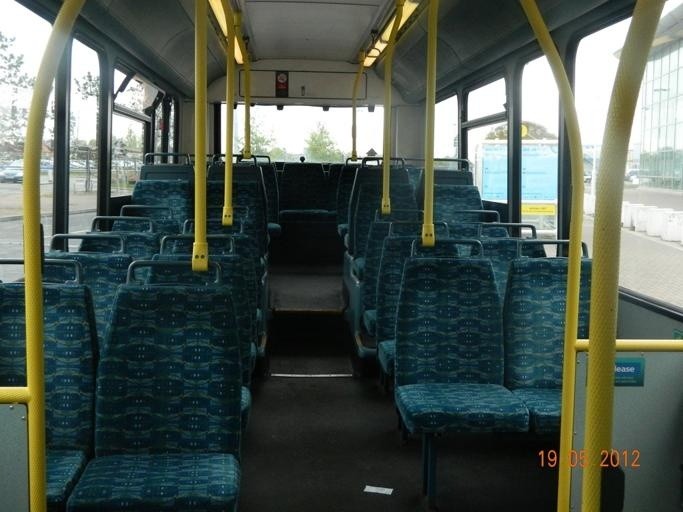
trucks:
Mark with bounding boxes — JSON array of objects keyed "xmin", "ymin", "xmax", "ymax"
[{"xmin": 475, "ymin": 139, "xmax": 559, "ymax": 239}]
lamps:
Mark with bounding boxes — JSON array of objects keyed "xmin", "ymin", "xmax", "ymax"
[
  {"xmin": 207, "ymin": 0, "xmax": 244, "ymax": 65},
  {"xmin": 363, "ymin": 0, "xmax": 423, "ymax": 69}
]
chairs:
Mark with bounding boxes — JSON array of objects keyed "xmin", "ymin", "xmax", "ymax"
[
  {"xmin": 277, "ymin": 158, "xmax": 335, "ymax": 255},
  {"xmin": 1, "ymin": 153, "xmax": 280, "ymax": 512},
  {"xmin": 326, "ymin": 158, "xmax": 592, "ymax": 503}
]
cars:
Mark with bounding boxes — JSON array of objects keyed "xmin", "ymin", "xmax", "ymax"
[{"xmin": 0, "ymin": 159, "xmax": 23, "ymax": 183}]
